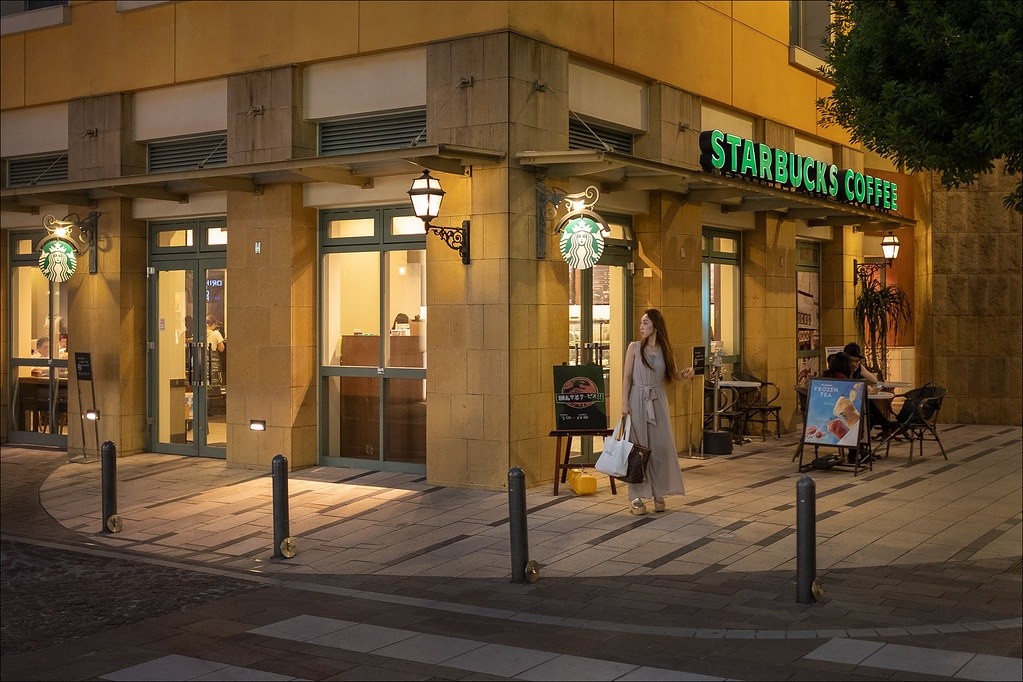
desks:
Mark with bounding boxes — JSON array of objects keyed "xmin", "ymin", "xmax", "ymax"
[
  {"xmin": 549, "ymin": 429, "xmax": 619, "ymax": 497},
  {"xmin": 19, "ymin": 377, "xmax": 69, "ymax": 435},
  {"xmin": 840, "ymin": 392, "xmax": 894, "ymax": 461},
  {"xmin": 717, "ymin": 382, "xmax": 761, "ymax": 444}
]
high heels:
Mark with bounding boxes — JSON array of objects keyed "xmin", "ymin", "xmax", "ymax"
[
  {"xmin": 628, "ymin": 497, "xmax": 646, "ymax": 515},
  {"xmin": 654, "ymin": 496, "xmax": 665, "ymax": 512}
]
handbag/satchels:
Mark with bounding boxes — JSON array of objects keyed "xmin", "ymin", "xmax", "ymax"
[
  {"xmin": 594, "ymin": 413, "xmax": 652, "ymax": 484},
  {"xmin": 896, "ymin": 382, "xmax": 938, "ymax": 424}
]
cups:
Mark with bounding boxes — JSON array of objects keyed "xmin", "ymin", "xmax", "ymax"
[
  {"xmin": 826, "ymin": 419, "xmax": 850, "ymax": 440},
  {"xmin": 834, "ymin": 399, "xmax": 860, "ymax": 430}
]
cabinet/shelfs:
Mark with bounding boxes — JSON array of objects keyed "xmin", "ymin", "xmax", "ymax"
[{"xmin": 568, "ymin": 318, "xmax": 610, "ymax": 395}]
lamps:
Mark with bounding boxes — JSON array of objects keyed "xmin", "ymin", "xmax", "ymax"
[
  {"xmin": 853, "ymin": 230, "xmax": 902, "ymax": 285},
  {"xmin": 406, "ymin": 168, "xmax": 470, "ymax": 265}
]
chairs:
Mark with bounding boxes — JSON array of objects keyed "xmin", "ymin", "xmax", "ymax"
[
  {"xmin": 793, "ymin": 384, "xmax": 949, "ymax": 471},
  {"xmin": 704, "ymin": 372, "xmax": 781, "ymax": 442}
]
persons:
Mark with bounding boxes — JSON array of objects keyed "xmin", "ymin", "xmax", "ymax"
[
  {"xmin": 183, "ymin": 314, "xmax": 225, "ymax": 388},
  {"xmin": 621, "ymin": 308, "xmax": 695, "ymax": 515},
  {"xmin": 31, "ymin": 333, "xmax": 69, "ymax": 426},
  {"xmin": 820, "ymin": 342, "xmax": 882, "ymax": 464}
]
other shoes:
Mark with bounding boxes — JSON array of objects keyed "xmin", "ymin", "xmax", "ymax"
[
  {"xmin": 882, "ymin": 421, "xmax": 909, "ymax": 440},
  {"xmin": 847, "ymin": 451, "xmax": 881, "ymax": 464}
]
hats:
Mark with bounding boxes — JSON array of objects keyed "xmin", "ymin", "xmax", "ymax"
[{"xmin": 844, "ymin": 343, "xmax": 864, "ymax": 359}]
